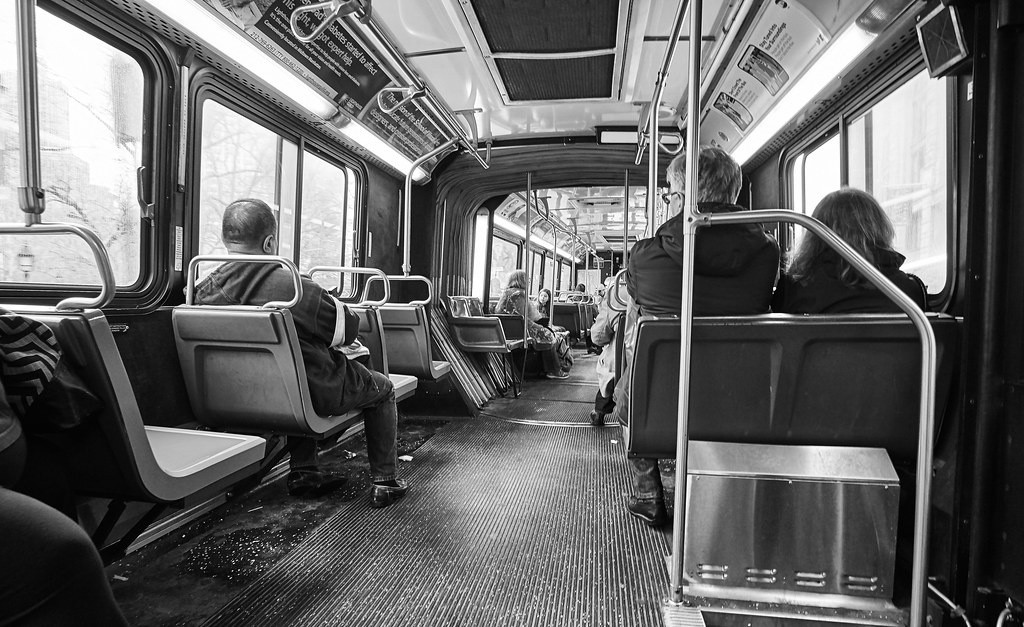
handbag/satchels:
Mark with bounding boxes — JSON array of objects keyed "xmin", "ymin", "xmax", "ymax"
[{"xmin": 329, "ymin": 294, "xmax": 370, "ymax": 362}]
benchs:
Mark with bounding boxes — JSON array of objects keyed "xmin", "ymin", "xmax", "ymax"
[{"xmin": 621, "ymin": 309, "xmax": 965, "ymax": 457}]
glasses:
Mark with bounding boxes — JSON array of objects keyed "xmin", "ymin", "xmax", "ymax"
[{"xmin": 662, "ymin": 191, "xmax": 676, "ymax": 205}]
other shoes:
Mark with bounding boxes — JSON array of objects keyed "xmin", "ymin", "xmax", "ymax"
[
  {"xmin": 627, "ymin": 495, "xmax": 668, "ymax": 527},
  {"xmin": 589, "ymin": 410, "xmax": 605, "ymax": 426},
  {"xmin": 369, "ymin": 477, "xmax": 408, "ymax": 508},
  {"xmin": 546, "ymin": 370, "xmax": 569, "ymax": 379}
]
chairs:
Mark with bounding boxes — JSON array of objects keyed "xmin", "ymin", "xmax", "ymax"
[
  {"xmin": 0, "ymin": 487, "xmax": 134, "ymax": 627},
  {"xmin": 0, "ymin": 223, "xmax": 266, "ymax": 568},
  {"xmin": 173, "ymin": 254, "xmax": 595, "ymax": 494}
]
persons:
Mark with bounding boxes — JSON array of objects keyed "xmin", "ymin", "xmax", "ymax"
[
  {"xmin": 769, "ymin": 187, "xmax": 929, "ymax": 315},
  {"xmin": 184, "ymin": 198, "xmax": 409, "ymax": 507},
  {"xmin": 613, "ymin": 144, "xmax": 779, "ymax": 524},
  {"xmin": 494, "ymin": 269, "xmax": 606, "ymax": 379},
  {"xmin": 590, "ymin": 252, "xmax": 630, "ymax": 426},
  {"xmin": 0, "ymin": 316, "xmax": 131, "ymax": 627}
]
